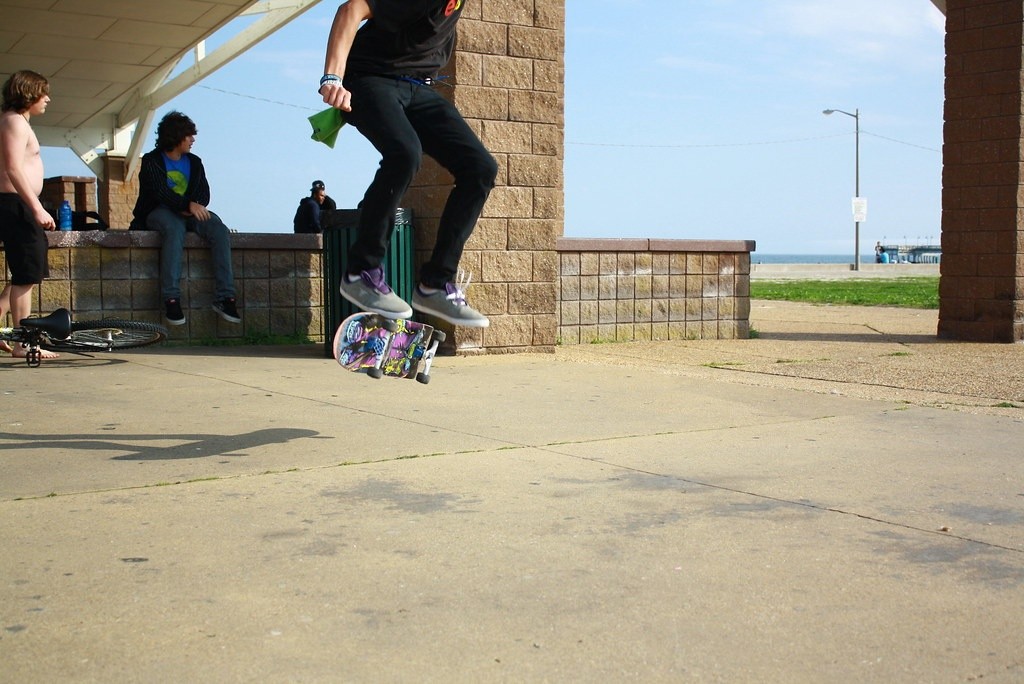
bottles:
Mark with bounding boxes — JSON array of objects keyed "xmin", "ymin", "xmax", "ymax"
[{"xmin": 60, "ymin": 200, "xmax": 72, "ymax": 231}]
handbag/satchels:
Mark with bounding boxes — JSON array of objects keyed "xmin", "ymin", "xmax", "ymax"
[{"xmin": 49, "ymin": 210, "xmax": 109, "ymax": 232}]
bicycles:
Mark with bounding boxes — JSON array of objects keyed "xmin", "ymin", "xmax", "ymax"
[{"xmin": 0, "ymin": 308, "xmax": 169, "ymax": 368}]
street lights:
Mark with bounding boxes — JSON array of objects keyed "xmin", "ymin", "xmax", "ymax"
[{"xmin": 822, "ymin": 108, "xmax": 860, "ymax": 271}]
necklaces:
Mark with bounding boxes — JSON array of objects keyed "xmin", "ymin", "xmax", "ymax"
[{"xmin": 21, "ymin": 112, "xmax": 30, "ymax": 125}]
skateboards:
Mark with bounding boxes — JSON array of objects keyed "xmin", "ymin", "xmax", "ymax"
[{"xmin": 331, "ymin": 311, "xmax": 447, "ymax": 385}]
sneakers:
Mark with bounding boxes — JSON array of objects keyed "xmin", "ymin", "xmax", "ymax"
[
  {"xmin": 411, "ymin": 269, "xmax": 489, "ymax": 329},
  {"xmin": 340, "ymin": 270, "xmax": 412, "ymax": 320},
  {"xmin": 212, "ymin": 295, "xmax": 240, "ymax": 323},
  {"xmin": 166, "ymin": 296, "xmax": 186, "ymax": 326}
]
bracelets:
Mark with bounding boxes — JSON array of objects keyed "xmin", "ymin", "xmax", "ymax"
[{"xmin": 318, "ymin": 75, "xmax": 344, "ymax": 93}]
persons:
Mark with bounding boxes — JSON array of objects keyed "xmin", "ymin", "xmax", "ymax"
[
  {"xmin": 319, "ymin": 0, "xmax": 499, "ymax": 329},
  {"xmin": 293, "ymin": 181, "xmax": 336, "ymax": 234},
  {"xmin": 129, "ymin": 110, "xmax": 242, "ymax": 325},
  {"xmin": 0, "ymin": 69, "xmax": 60, "ymax": 358}
]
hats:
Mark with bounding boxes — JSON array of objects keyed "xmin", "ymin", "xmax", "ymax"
[{"xmin": 310, "ymin": 180, "xmax": 324, "ymax": 191}]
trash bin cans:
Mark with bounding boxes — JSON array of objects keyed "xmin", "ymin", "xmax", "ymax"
[{"xmin": 321, "ymin": 207, "xmax": 414, "ymax": 359}]
